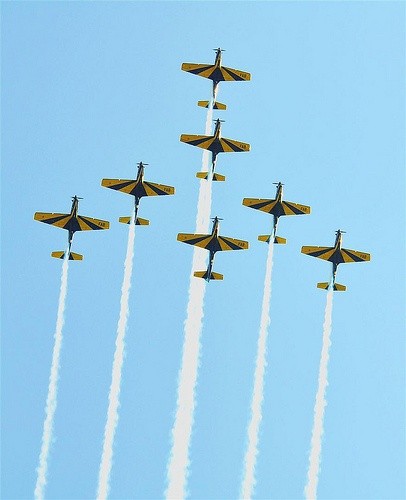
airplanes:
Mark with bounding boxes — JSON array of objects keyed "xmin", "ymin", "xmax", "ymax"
[
  {"xmin": 34, "ymin": 195, "xmax": 109, "ymax": 261},
  {"xmin": 242, "ymin": 181, "xmax": 310, "ymax": 245},
  {"xmin": 181, "ymin": 48, "xmax": 251, "ymax": 110},
  {"xmin": 301, "ymin": 229, "xmax": 370, "ymax": 291},
  {"xmin": 101, "ymin": 162, "xmax": 175, "ymax": 227},
  {"xmin": 179, "ymin": 118, "xmax": 250, "ymax": 181},
  {"xmin": 177, "ymin": 216, "xmax": 250, "ymax": 283}
]
